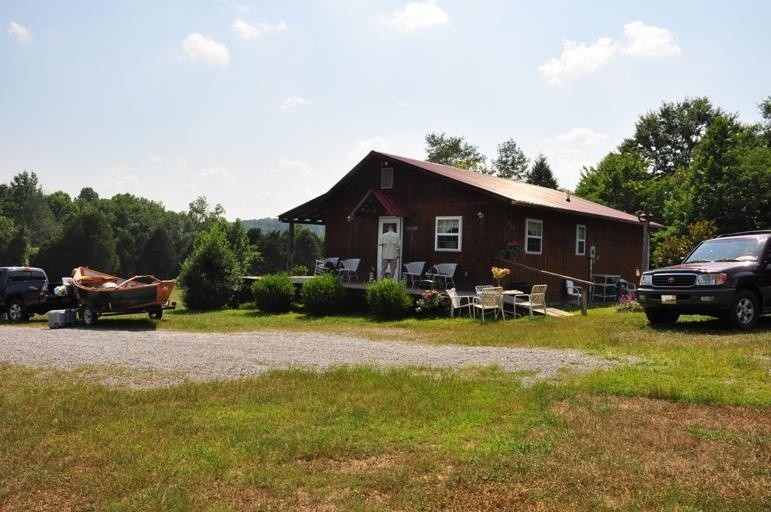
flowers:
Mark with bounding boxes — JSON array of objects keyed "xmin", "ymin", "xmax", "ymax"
[
  {"xmin": 503, "ymin": 240, "xmax": 527, "ymax": 261},
  {"xmin": 490, "ymin": 265, "xmax": 511, "ymax": 280}
]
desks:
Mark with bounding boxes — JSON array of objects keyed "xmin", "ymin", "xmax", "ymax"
[{"xmin": 591, "ymin": 273, "xmax": 621, "ymax": 304}]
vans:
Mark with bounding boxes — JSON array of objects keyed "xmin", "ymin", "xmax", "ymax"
[{"xmin": 0, "ymin": 263, "xmax": 49, "ymax": 323}]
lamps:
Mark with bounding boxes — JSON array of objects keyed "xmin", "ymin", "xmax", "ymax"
[{"xmin": 477, "ymin": 211, "xmax": 484, "ymax": 219}]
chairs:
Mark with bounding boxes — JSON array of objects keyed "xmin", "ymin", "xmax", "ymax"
[
  {"xmin": 311, "ymin": 255, "xmax": 363, "ymax": 283},
  {"xmin": 401, "ymin": 261, "xmax": 459, "ymax": 294},
  {"xmin": 618, "ymin": 278, "xmax": 637, "ymax": 298},
  {"xmin": 565, "ymin": 278, "xmax": 583, "ymax": 308},
  {"xmin": 444, "ymin": 280, "xmax": 551, "ymax": 322}
]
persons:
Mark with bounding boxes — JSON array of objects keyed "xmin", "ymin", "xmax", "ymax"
[{"xmin": 378, "ymin": 226, "xmax": 401, "ymax": 280}]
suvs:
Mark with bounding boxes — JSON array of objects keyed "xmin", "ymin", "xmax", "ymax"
[{"xmin": 630, "ymin": 228, "xmax": 770, "ymax": 330}]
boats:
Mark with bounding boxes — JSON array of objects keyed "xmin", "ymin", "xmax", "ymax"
[{"xmin": 67, "ymin": 265, "xmax": 177, "ymax": 326}]
